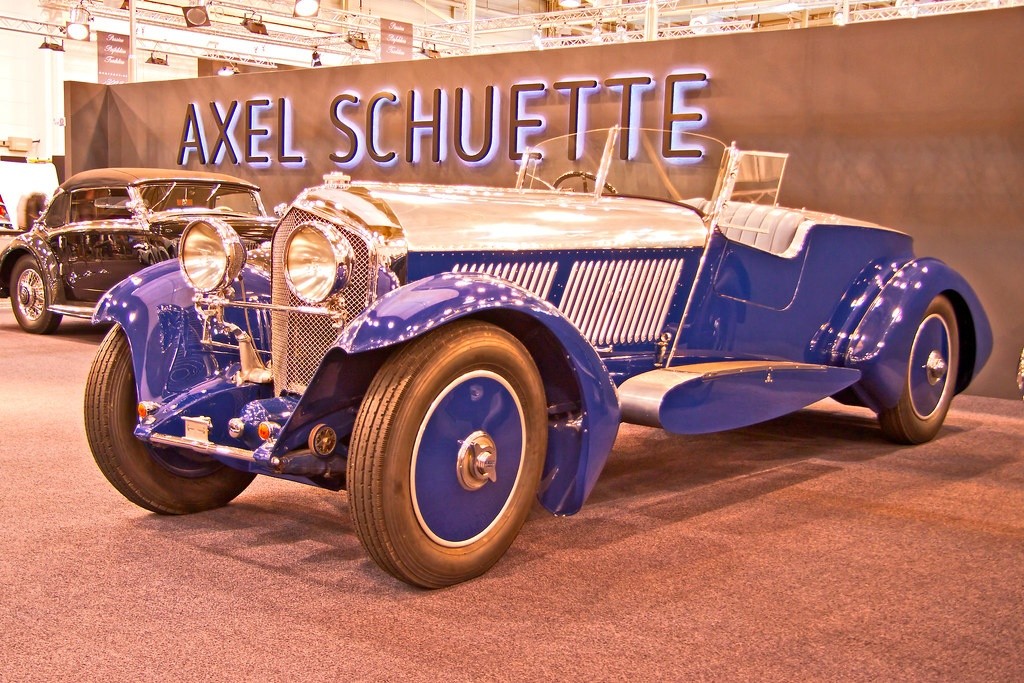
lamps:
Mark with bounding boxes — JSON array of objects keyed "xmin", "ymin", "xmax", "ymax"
[{"xmin": 38, "ymin": 0, "xmax": 441, "ymax": 68}]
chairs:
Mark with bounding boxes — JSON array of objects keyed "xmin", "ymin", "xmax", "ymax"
[{"xmin": 704, "ymin": 202, "xmax": 804, "ymax": 255}]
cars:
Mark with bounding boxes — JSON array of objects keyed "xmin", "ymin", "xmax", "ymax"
[
  {"xmin": 0, "ymin": 167, "xmax": 283, "ymax": 335},
  {"xmin": 80, "ymin": 125, "xmax": 995, "ymax": 590}
]
